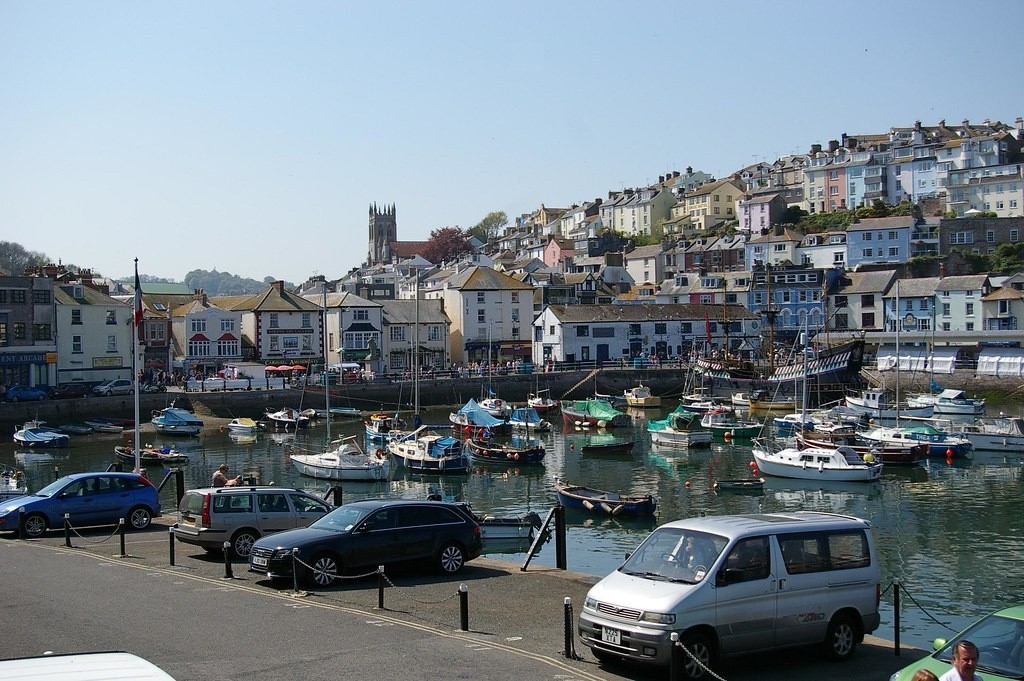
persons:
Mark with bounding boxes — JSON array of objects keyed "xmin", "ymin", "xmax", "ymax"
[
  {"xmin": 211, "ymin": 463, "xmax": 244, "ymax": 486},
  {"xmin": 140, "ymin": 368, "xmax": 222, "ymax": 389},
  {"xmin": 911, "ymin": 640, "xmax": 984, "ymax": 681},
  {"xmin": 678, "ymin": 536, "xmax": 705, "ymax": 571},
  {"xmin": 467, "ymin": 357, "xmax": 523, "ymax": 375},
  {"xmin": 640, "ymin": 352, "xmax": 659, "ymax": 369},
  {"xmin": 0, "ymin": 384, "xmax": 6, "ymax": 403},
  {"xmin": 298, "ymin": 369, "xmax": 364, "ymax": 384}
]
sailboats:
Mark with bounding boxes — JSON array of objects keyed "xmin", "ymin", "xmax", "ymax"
[
  {"xmin": 288, "ymin": 284, "xmax": 389, "ymax": 481},
  {"xmin": 677, "ymin": 264, "xmax": 988, "ymax": 482},
  {"xmin": 386, "ymin": 266, "xmax": 561, "ymax": 474}
]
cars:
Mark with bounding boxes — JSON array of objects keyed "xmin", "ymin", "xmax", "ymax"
[
  {"xmin": 0, "ymin": 471, "xmax": 162, "ymax": 539},
  {"xmin": 890, "ymin": 604, "xmax": 1023, "ymax": 681},
  {"xmin": 0, "ymin": 378, "xmax": 134, "ymax": 399},
  {"xmin": 248, "ymin": 500, "xmax": 485, "ymax": 589}
]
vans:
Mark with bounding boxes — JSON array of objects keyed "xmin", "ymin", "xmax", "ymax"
[{"xmin": 575, "ymin": 505, "xmax": 882, "ymax": 681}]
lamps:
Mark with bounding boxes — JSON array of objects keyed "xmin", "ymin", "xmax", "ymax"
[
  {"xmin": 342, "ymin": 306, "xmax": 351, "ymax": 313},
  {"xmin": 126, "ymin": 318, "xmax": 132, "ymax": 325}
]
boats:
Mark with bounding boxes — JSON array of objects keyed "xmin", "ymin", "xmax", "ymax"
[
  {"xmin": 363, "ymin": 412, "xmax": 406, "ymax": 442},
  {"xmin": 228, "ymin": 417, "xmax": 266, "ymax": 432},
  {"xmin": 508, "ymin": 408, "xmax": 554, "ymax": 433},
  {"xmin": 475, "ymin": 384, "xmax": 929, "ymax": 540},
  {"xmin": 328, "ymin": 407, "xmax": 362, "ymax": 418},
  {"xmin": 13, "ymin": 410, "xmax": 135, "ymax": 448},
  {"xmin": 150, "ymin": 406, "xmax": 204, "ymax": 436},
  {"xmin": 14, "ymin": 450, "xmax": 54, "ymax": 466},
  {"xmin": 265, "ymin": 407, "xmax": 310, "ymax": 429},
  {"xmin": 0, "ymin": 463, "xmax": 28, "ymax": 502},
  {"xmin": 114, "ymin": 445, "xmax": 186, "ymax": 465},
  {"xmin": 448, "ymin": 398, "xmax": 512, "ymax": 434},
  {"xmin": 465, "ymin": 432, "xmax": 545, "ymax": 465}
]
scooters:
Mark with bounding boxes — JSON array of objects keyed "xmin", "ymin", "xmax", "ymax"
[{"xmin": 139, "ymin": 379, "xmax": 167, "ymax": 394}]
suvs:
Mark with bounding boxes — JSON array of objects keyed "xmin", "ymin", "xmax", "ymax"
[{"xmin": 173, "ymin": 489, "xmax": 338, "ymax": 562}]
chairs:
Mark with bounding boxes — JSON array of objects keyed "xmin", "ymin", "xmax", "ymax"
[
  {"xmin": 79, "ymin": 481, "xmax": 88, "ymax": 496},
  {"xmin": 784, "ymin": 542, "xmax": 803, "ymax": 563},
  {"xmin": 261, "ymin": 495, "xmax": 274, "ymax": 512},
  {"xmin": 274, "ymin": 497, "xmax": 289, "ymax": 512},
  {"xmin": 88, "ymin": 483, "xmax": 95, "ymax": 495},
  {"xmin": 699, "ymin": 538, "xmax": 718, "ymax": 569},
  {"xmin": 104, "ymin": 480, "xmax": 117, "ymax": 492}
]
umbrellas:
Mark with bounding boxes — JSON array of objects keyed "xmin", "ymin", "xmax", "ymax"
[
  {"xmin": 218, "ymin": 368, "xmax": 233, "ymax": 373},
  {"xmin": 266, "ymin": 365, "xmax": 307, "ymax": 377}
]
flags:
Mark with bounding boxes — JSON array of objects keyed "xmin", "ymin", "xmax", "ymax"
[{"xmin": 134, "ymin": 273, "xmax": 143, "ymax": 327}]
